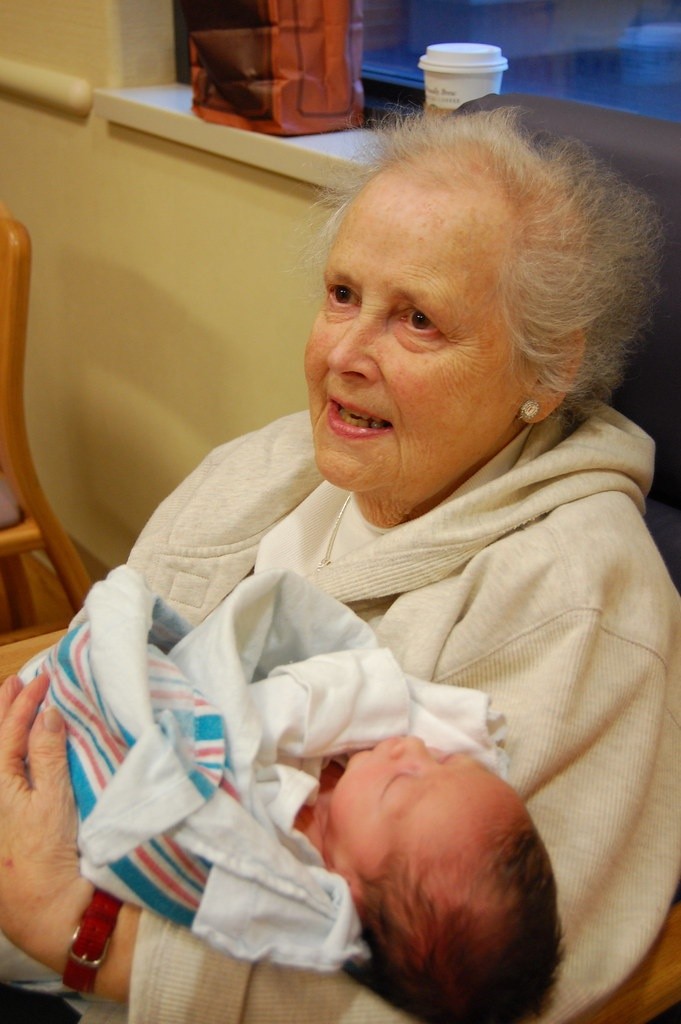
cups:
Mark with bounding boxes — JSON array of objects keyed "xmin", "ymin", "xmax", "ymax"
[{"xmin": 418, "ymin": 43, "xmax": 507, "ymax": 125}]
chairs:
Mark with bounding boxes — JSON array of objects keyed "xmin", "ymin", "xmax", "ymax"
[{"xmin": 0, "ymin": 221, "xmax": 94, "ymax": 645}]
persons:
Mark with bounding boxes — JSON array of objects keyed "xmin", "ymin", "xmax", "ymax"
[{"xmin": 0, "ymin": 112, "xmax": 681, "ymax": 1024}]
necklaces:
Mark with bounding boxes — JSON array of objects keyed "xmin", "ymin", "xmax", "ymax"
[{"xmin": 316, "ymin": 492, "xmax": 352, "ymax": 570}]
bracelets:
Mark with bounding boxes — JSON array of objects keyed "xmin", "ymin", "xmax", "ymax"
[{"xmin": 63, "ymin": 887, "xmax": 122, "ymax": 993}]
